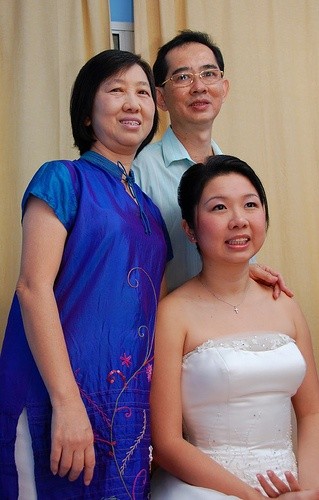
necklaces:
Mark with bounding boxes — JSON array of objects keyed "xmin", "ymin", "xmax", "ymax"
[{"xmin": 201, "ymin": 271, "xmax": 251, "ymax": 314}]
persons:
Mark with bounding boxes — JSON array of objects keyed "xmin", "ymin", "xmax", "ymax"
[
  {"xmin": 0, "ymin": 50, "xmax": 294, "ymax": 500},
  {"xmin": 132, "ymin": 29, "xmax": 258, "ymax": 293},
  {"xmin": 149, "ymin": 154, "xmax": 319, "ymax": 500}
]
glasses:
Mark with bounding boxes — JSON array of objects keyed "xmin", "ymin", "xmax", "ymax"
[{"xmin": 160, "ymin": 70, "xmax": 223, "ymax": 88}]
